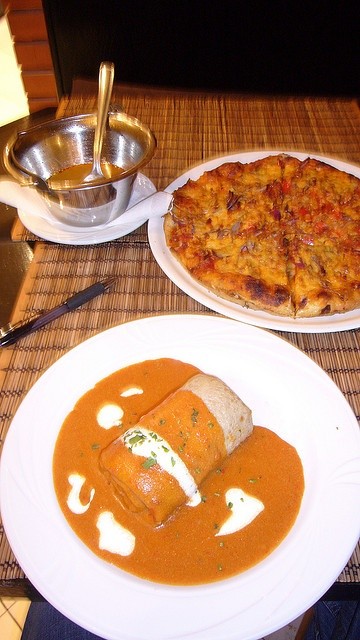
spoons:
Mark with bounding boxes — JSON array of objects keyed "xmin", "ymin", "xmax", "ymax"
[{"xmin": 82, "ymin": 58, "xmax": 119, "ymax": 185}]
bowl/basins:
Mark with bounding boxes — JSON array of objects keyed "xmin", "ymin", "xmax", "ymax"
[
  {"xmin": 146, "ymin": 147, "xmax": 359, "ymax": 335},
  {"xmin": 3, "ymin": 113, "xmax": 154, "ymax": 229}
]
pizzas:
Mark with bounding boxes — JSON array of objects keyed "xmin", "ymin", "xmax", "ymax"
[{"xmin": 163, "ymin": 152, "xmax": 359, "ymax": 319}]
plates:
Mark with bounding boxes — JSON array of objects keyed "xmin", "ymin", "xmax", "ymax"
[
  {"xmin": 16, "ymin": 177, "xmax": 162, "ymax": 250},
  {"xmin": 0, "ymin": 315, "xmax": 358, "ymax": 640}
]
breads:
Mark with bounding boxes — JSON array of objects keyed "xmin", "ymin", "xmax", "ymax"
[{"xmin": 98, "ymin": 373, "xmax": 252, "ymax": 527}]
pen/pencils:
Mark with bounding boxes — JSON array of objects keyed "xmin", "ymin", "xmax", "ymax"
[{"xmin": 0, "ymin": 277, "xmax": 119, "ymax": 349}]
